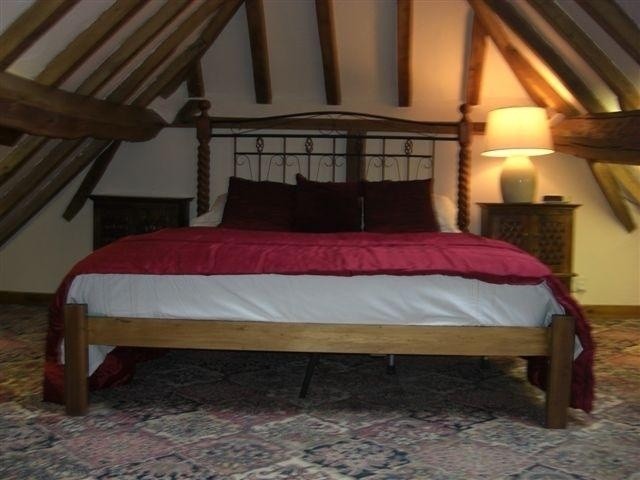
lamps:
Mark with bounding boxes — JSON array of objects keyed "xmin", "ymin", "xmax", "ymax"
[{"xmin": 480, "ymin": 107, "xmax": 556, "ymax": 203}]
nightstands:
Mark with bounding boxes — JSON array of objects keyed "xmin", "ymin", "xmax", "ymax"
[
  {"xmin": 87, "ymin": 193, "xmax": 194, "ymax": 251},
  {"xmin": 477, "ymin": 200, "xmax": 582, "ymax": 294}
]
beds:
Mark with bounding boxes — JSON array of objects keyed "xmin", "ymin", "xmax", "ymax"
[{"xmin": 42, "ymin": 99, "xmax": 596, "ymax": 431}]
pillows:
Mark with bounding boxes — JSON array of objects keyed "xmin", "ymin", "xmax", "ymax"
[
  {"xmin": 294, "ymin": 173, "xmax": 363, "ymax": 233},
  {"xmin": 216, "ymin": 177, "xmax": 296, "ymax": 231},
  {"xmin": 358, "ymin": 179, "xmax": 442, "ymax": 234}
]
